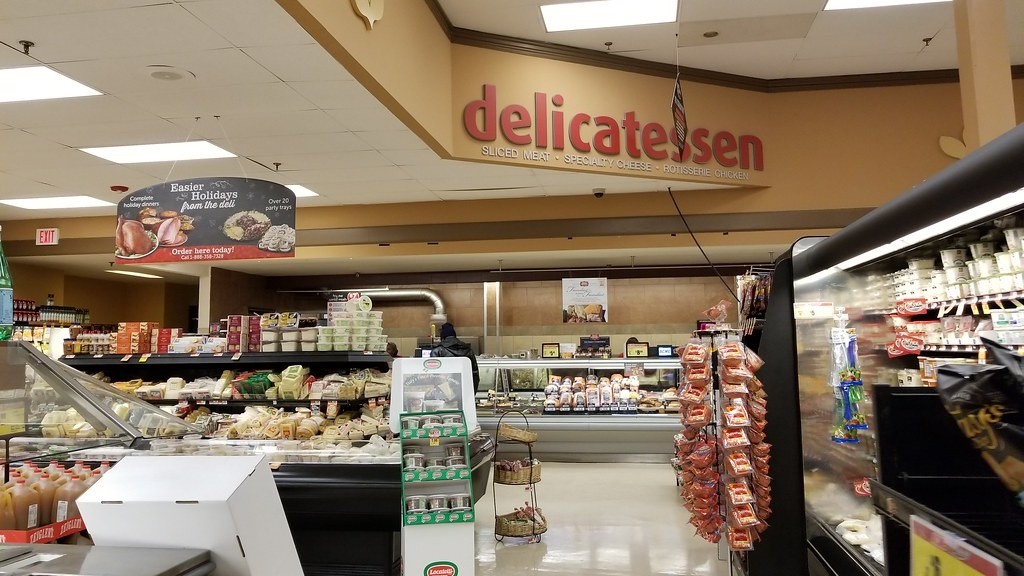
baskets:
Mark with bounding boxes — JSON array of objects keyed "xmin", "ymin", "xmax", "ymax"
[
  {"xmin": 498, "ymin": 423, "xmax": 538, "ymax": 442},
  {"xmin": 496, "ymin": 511, "xmax": 547, "ymax": 536},
  {"xmin": 495, "ymin": 462, "xmax": 541, "ymax": 484}
]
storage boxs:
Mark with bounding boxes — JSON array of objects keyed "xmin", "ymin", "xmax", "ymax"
[
  {"xmin": 117, "ymin": 314, "xmax": 263, "ymax": 354},
  {"xmin": 74, "ymin": 454, "xmax": 306, "ymax": 576}
]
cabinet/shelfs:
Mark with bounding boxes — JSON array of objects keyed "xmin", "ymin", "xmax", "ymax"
[
  {"xmin": 890, "ymin": 288, "xmax": 1024, "ymax": 353},
  {"xmin": 399, "ymin": 410, "xmax": 475, "ymax": 576},
  {"xmin": 57, "ymin": 351, "xmax": 394, "ymax": 419}
]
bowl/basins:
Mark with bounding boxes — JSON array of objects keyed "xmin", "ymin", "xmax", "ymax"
[{"xmin": 402, "ymin": 414, "xmax": 472, "ymax": 514}]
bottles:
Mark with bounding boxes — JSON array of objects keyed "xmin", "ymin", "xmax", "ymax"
[
  {"xmin": 36, "ymin": 305, "xmax": 89, "ymax": 323},
  {"xmin": 0, "ymin": 225, "xmax": 13, "ymax": 341},
  {"xmin": 0, "ymin": 458, "xmax": 110, "ymax": 530}
]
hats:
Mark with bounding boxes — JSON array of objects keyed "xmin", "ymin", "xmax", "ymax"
[{"xmin": 440, "ymin": 322, "xmax": 456, "ymax": 339}]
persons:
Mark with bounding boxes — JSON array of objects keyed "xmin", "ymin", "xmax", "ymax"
[
  {"xmin": 428, "ymin": 322, "xmax": 480, "ymax": 397},
  {"xmin": 386, "ymin": 342, "xmax": 401, "ymax": 358}
]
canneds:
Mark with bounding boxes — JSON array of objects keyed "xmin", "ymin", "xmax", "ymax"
[{"xmin": 13, "ymin": 297, "xmax": 40, "ymax": 322}]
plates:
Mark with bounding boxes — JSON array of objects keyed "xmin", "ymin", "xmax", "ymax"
[{"xmin": 116, "ymin": 209, "xmax": 194, "ymax": 260}]
